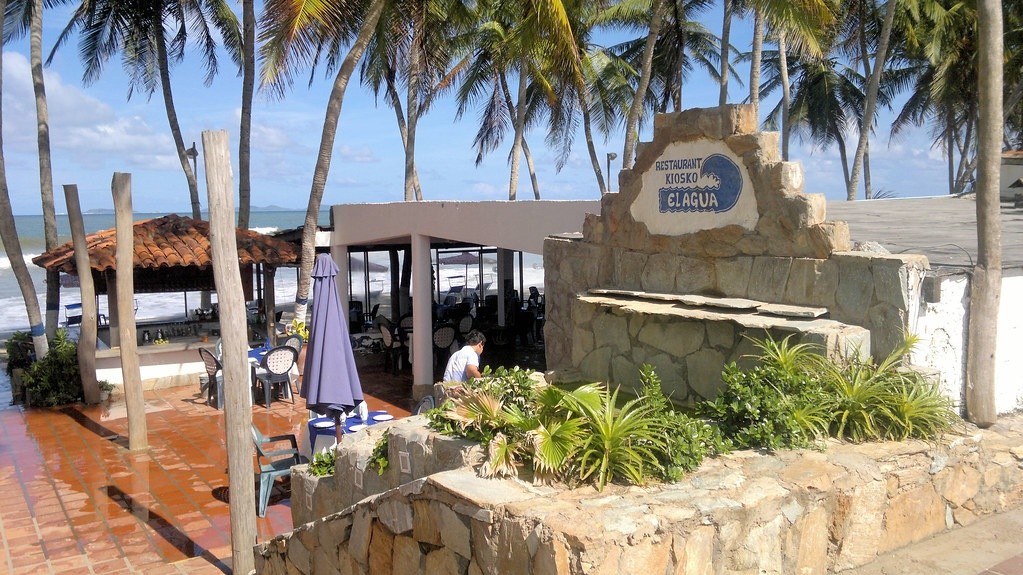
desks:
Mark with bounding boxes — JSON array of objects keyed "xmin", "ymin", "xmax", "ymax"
[
  {"xmin": 217, "ymin": 346, "xmax": 280, "ymax": 401},
  {"xmin": 303, "ymin": 411, "xmax": 395, "ymax": 465}
]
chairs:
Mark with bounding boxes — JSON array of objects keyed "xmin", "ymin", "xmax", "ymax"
[
  {"xmin": 59, "ymin": 303, "xmax": 82, "ymax": 327},
  {"xmin": 410, "ymin": 395, "xmax": 435, "ymax": 415},
  {"xmin": 379, "ymin": 274, "xmax": 545, "ymax": 381},
  {"xmin": 362, "ymin": 304, "xmax": 380, "ymax": 325},
  {"xmin": 199, "ymin": 334, "xmax": 303, "ymax": 409},
  {"xmin": 251, "ymin": 421, "xmax": 302, "ymax": 518}
]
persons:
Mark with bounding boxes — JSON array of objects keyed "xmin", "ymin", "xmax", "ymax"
[{"xmin": 443, "ymin": 330, "xmax": 486, "ymax": 382}]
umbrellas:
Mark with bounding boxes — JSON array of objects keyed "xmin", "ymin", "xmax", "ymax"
[
  {"xmin": 346, "ymin": 257, "xmax": 388, "ymax": 273},
  {"xmin": 439, "ymin": 253, "xmax": 497, "ymax": 295},
  {"xmin": 306, "ymin": 254, "xmax": 364, "ymax": 444}
]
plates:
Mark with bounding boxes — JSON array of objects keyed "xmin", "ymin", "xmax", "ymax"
[
  {"xmin": 349, "ymin": 424, "xmax": 368, "ymax": 431},
  {"xmin": 313, "ymin": 421, "xmax": 335, "ymax": 428},
  {"xmin": 259, "ymin": 352, "xmax": 267, "ymax": 355},
  {"xmin": 347, "ymin": 413, "xmax": 357, "ymax": 418},
  {"xmin": 372, "ymin": 414, "xmax": 394, "ymax": 421}
]
